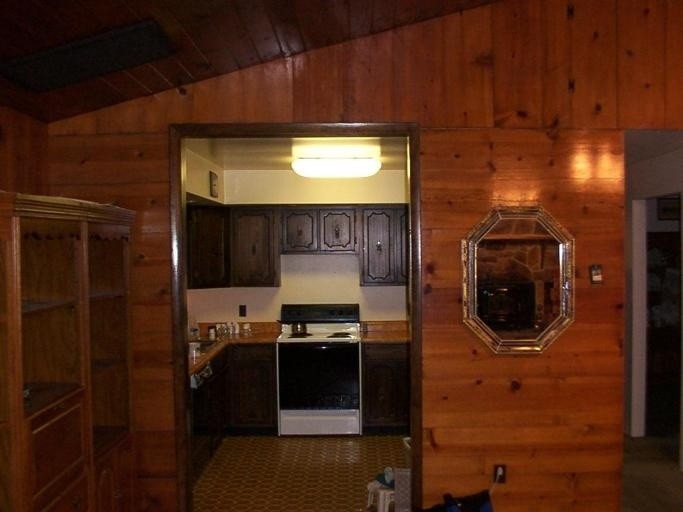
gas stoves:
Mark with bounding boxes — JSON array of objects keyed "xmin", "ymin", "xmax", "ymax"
[{"xmin": 277, "ymin": 324, "xmax": 362, "ymax": 344}]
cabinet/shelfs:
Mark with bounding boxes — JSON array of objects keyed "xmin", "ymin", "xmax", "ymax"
[
  {"xmin": 185, "ymin": 201, "xmax": 280, "ymax": 290},
  {"xmin": 362, "ymin": 339, "xmax": 410, "ymax": 427},
  {"xmin": 281, "ymin": 205, "xmax": 359, "ymax": 255},
  {"xmin": 226, "ymin": 337, "xmax": 277, "ymax": 429},
  {"xmin": 0, "ymin": 190, "xmax": 138, "ymax": 512},
  {"xmin": 190, "ymin": 351, "xmax": 226, "ymax": 479},
  {"xmin": 359, "ymin": 204, "xmax": 409, "ymax": 286}
]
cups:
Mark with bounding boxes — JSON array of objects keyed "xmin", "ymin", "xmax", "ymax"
[{"xmin": 187, "ymin": 341, "xmax": 202, "ymax": 357}]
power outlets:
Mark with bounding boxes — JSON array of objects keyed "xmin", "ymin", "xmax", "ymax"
[{"xmin": 493, "ymin": 464, "xmax": 506, "ymax": 484}]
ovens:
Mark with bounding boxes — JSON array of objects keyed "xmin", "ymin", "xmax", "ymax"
[{"xmin": 276, "ymin": 345, "xmax": 363, "ymax": 433}]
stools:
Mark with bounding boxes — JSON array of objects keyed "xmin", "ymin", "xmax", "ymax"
[{"xmin": 367, "ymin": 487, "xmax": 395, "ymax": 512}]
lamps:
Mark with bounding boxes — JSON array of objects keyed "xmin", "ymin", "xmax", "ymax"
[
  {"xmin": 292, "ymin": 158, "xmax": 382, "ymax": 179},
  {"xmin": 292, "ymin": 143, "xmax": 381, "ymax": 159}
]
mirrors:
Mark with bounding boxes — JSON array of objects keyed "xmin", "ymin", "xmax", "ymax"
[{"xmin": 460, "ymin": 202, "xmax": 576, "ymax": 355}]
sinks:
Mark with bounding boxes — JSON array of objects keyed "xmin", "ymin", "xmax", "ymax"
[{"xmin": 188, "ymin": 341, "xmax": 217, "ymax": 359}]
